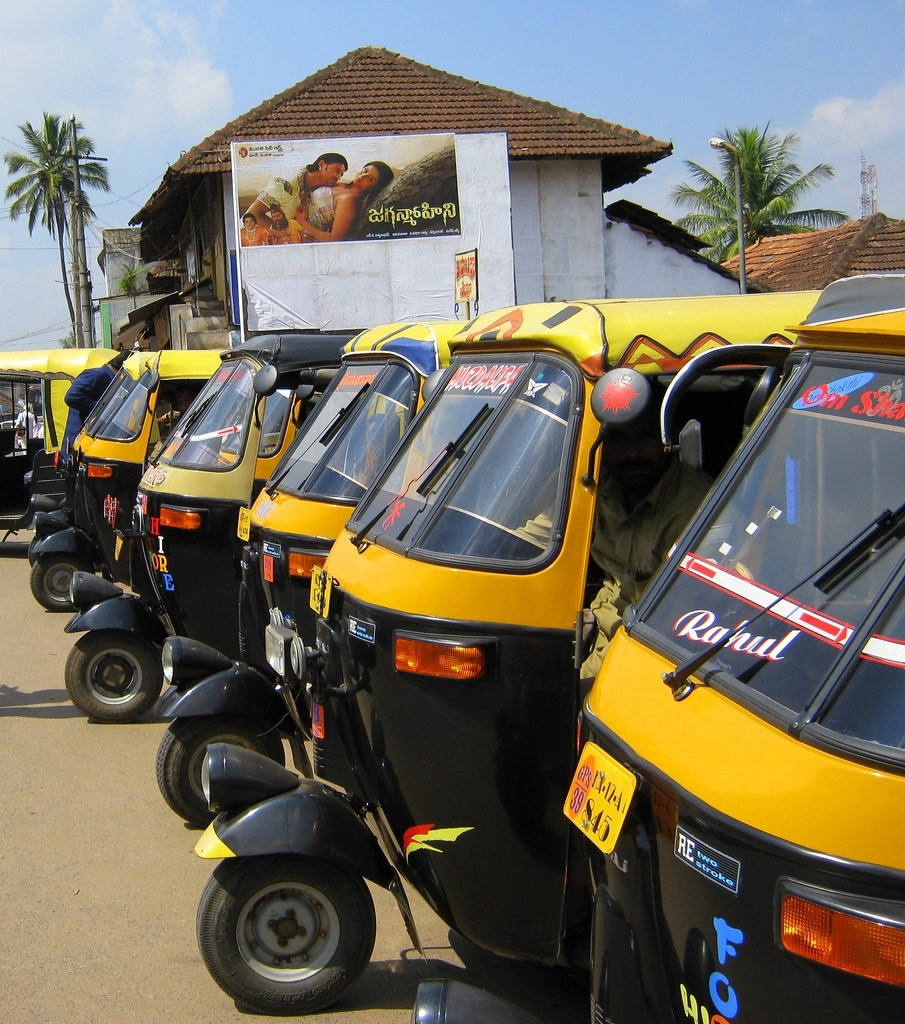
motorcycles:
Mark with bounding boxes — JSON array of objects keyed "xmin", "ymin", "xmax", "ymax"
[{"xmin": 1, "ymin": 266, "xmax": 905, "ymax": 1024}]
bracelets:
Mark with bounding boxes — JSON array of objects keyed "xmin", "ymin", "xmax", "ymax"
[{"xmin": 297, "ymin": 217, "xmax": 309, "ymax": 221}]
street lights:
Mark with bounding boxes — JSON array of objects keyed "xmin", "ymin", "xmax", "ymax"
[{"xmin": 710, "ymin": 137, "xmax": 748, "ymax": 295}]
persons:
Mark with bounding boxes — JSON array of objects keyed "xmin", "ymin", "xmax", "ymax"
[
  {"xmin": 15, "ymin": 404, "xmax": 35, "ymax": 454},
  {"xmin": 58, "ymin": 350, "xmax": 135, "ymax": 507},
  {"xmin": 268, "ymin": 207, "xmax": 304, "ymax": 245},
  {"xmin": 239, "ymin": 213, "xmax": 269, "ymax": 247},
  {"xmin": 294, "ymin": 160, "xmax": 394, "ymax": 242},
  {"xmin": 511, "ymin": 394, "xmax": 715, "ymax": 706},
  {"xmin": 146, "ymin": 383, "xmax": 201, "ymax": 469},
  {"xmin": 241, "ymin": 152, "xmax": 348, "ymax": 229}
]
